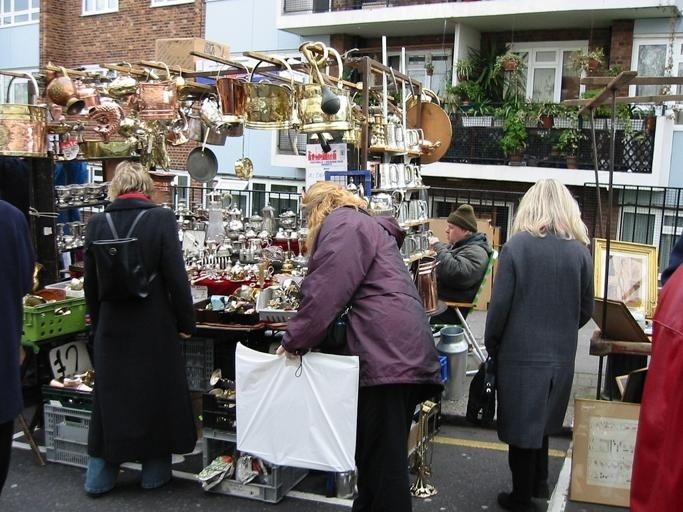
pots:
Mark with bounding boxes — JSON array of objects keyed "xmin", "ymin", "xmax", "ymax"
[
  {"xmin": 186, "ymin": 126, "xmax": 218, "ymax": 183},
  {"xmin": 234, "ymin": 134, "xmax": 253, "ymax": 181},
  {"xmin": 226, "ymin": 121, "xmax": 244, "ymax": 137},
  {"xmin": 182, "ymin": 112, "xmax": 228, "ymax": 146}
]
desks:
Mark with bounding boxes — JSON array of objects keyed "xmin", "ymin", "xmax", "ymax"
[
  {"xmin": 33, "ymin": 281, "xmax": 449, "ymax": 498},
  {"xmin": 588, "ymin": 330, "xmax": 653, "ymax": 399}
]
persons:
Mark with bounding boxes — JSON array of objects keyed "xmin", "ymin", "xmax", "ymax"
[
  {"xmin": 483, "ymin": 178, "xmax": 594, "ymax": 511},
  {"xmin": 0, "ymin": 197, "xmax": 36, "ymax": 489},
  {"xmin": 274, "ymin": 181, "xmax": 444, "ymax": 512},
  {"xmin": 628, "ymin": 236, "xmax": 683, "ymax": 511},
  {"xmin": 54, "ymin": 156, "xmax": 88, "ymax": 234},
  {"xmin": 81, "ymin": 160, "xmax": 198, "ymax": 499},
  {"xmin": 428, "ymin": 202, "xmax": 491, "ymax": 324}
]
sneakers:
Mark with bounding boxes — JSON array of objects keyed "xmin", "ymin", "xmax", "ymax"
[{"xmin": 496, "ymin": 490, "xmax": 549, "ymax": 512}]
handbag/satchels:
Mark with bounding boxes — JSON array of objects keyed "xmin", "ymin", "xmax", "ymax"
[
  {"xmin": 466, "ymin": 355, "xmax": 498, "ymax": 429},
  {"xmin": 234, "ymin": 341, "xmax": 361, "ymax": 474}
]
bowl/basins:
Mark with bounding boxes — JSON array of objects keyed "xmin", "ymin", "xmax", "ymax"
[
  {"xmin": 45, "ymin": 121, "xmax": 71, "ymax": 135},
  {"xmin": 76, "ymin": 139, "xmax": 105, "ymax": 158}
]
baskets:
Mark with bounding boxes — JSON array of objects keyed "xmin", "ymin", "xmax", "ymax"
[{"xmin": 21, "ymin": 296, "xmax": 88, "ymax": 343}]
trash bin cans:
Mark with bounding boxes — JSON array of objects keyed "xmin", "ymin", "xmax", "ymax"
[{"xmin": 436, "ymin": 325, "xmax": 469, "ymax": 402}]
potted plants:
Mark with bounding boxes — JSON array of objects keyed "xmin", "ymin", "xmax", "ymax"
[
  {"xmin": 494, "ymin": 115, "xmax": 528, "ymax": 165},
  {"xmin": 643, "ymin": 108, "xmax": 656, "ymax": 137},
  {"xmin": 554, "ymin": 129, "xmax": 590, "ymax": 169},
  {"xmin": 562, "ymin": 46, "xmax": 604, "ymax": 70},
  {"xmin": 424, "ymin": 63, "xmax": 434, "ymax": 75},
  {"xmin": 535, "ymin": 101, "xmax": 557, "ymax": 129},
  {"xmin": 446, "ymin": 81, "xmax": 487, "ymax": 105},
  {"xmin": 456, "ymin": 58, "xmax": 473, "ymax": 82},
  {"xmin": 490, "ymin": 53, "xmax": 521, "ymax": 78}
]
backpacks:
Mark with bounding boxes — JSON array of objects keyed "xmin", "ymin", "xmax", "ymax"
[{"xmin": 91, "ymin": 209, "xmax": 158, "ymax": 305}]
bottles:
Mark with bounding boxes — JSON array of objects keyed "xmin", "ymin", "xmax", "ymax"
[{"xmin": 334, "ymin": 469, "xmax": 356, "ymax": 499}]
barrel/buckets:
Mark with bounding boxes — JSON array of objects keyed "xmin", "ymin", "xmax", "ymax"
[
  {"xmin": 298, "ymin": 60, "xmax": 350, "ymax": 134},
  {"xmin": 213, "ymin": 63, "xmax": 249, "ymax": 115},
  {"xmin": 138, "ymin": 62, "xmax": 177, "ymax": 119},
  {"xmin": 247, "ymin": 56, "xmax": 293, "ymax": 130},
  {"xmin": 347, "ymin": 88, "xmax": 386, "ymax": 147},
  {"xmin": 412, "ymin": 257, "xmax": 439, "ymax": 313},
  {"xmin": 1, "ymin": 71, "xmax": 50, "ymax": 162}
]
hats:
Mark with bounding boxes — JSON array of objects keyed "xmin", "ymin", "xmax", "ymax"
[{"xmin": 447, "ymin": 204, "xmax": 478, "ymax": 234}]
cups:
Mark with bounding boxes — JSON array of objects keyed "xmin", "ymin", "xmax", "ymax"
[
  {"xmin": 386, "ymin": 122, "xmax": 425, "ymax": 151},
  {"xmin": 398, "ymin": 229, "xmax": 434, "ymax": 259},
  {"xmin": 395, "ymin": 199, "xmax": 428, "ymax": 223},
  {"xmin": 374, "ymin": 162, "xmax": 422, "ymax": 189}
]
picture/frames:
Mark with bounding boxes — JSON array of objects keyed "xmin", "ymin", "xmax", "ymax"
[
  {"xmin": 571, "ymin": 398, "xmax": 642, "ymax": 507},
  {"xmin": 615, "ymin": 374, "xmax": 627, "ymax": 396},
  {"xmin": 620, "ymin": 366, "xmax": 648, "ymax": 404},
  {"xmin": 592, "ymin": 237, "xmax": 658, "ymax": 318},
  {"xmin": 590, "ymin": 297, "xmax": 650, "ymax": 342}
]
